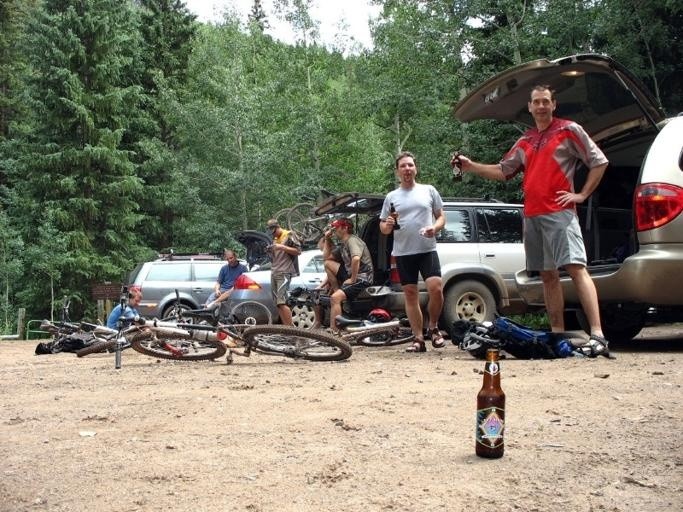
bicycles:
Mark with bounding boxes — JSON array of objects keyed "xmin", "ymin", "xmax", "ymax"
[
  {"xmin": 79, "ymin": 287, "xmax": 187, "ymax": 357},
  {"xmin": 273, "ymin": 207, "xmax": 324, "ymax": 241},
  {"xmin": 285, "ymin": 195, "xmax": 367, "ymax": 245},
  {"xmin": 39, "ymin": 317, "xmax": 119, "ymax": 341},
  {"xmin": 115, "ymin": 286, "xmax": 352, "ymax": 369},
  {"xmin": 332, "ymin": 315, "xmax": 414, "ymax": 347}
]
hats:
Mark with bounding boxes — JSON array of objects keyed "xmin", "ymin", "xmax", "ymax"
[
  {"xmin": 266, "ymin": 219, "xmax": 278, "ymax": 235},
  {"xmin": 331, "ymin": 218, "xmax": 353, "ymax": 229}
]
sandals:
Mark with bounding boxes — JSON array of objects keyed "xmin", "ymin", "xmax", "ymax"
[
  {"xmin": 574, "ymin": 337, "xmax": 610, "ymax": 359},
  {"xmin": 427, "ymin": 327, "xmax": 445, "ymax": 347},
  {"xmin": 405, "ymin": 336, "xmax": 426, "ymax": 353}
]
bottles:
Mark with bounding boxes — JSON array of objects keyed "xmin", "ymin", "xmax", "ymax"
[
  {"xmin": 452, "ymin": 151, "xmax": 462, "ymax": 182},
  {"xmin": 390, "ymin": 203, "xmax": 400, "ymax": 230},
  {"xmin": 475, "ymin": 348, "xmax": 506, "ymax": 460}
]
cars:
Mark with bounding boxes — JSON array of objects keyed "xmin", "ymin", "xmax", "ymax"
[
  {"xmin": 453, "ymin": 54, "xmax": 683, "ymax": 344},
  {"xmin": 230, "ymin": 249, "xmax": 327, "ymax": 330}
]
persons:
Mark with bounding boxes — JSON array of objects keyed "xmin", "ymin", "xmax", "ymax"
[
  {"xmin": 204, "ymin": 250, "xmax": 248, "ymax": 308},
  {"xmin": 107, "ymin": 288, "xmax": 142, "ymax": 330},
  {"xmin": 324, "ymin": 217, "xmax": 373, "ymax": 335},
  {"xmin": 450, "ymin": 83, "xmax": 609, "ymax": 355},
  {"xmin": 378, "ymin": 151, "xmax": 447, "ymax": 353},
  {"xmin": 310, "ymin": 234, "xmax": 341, "ymax": 330},
  {"xmin": 263, "ymin": 219, "xmax": 301, "ymax": 327}
]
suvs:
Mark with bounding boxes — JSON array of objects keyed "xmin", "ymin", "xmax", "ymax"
[
  {"xmin": 126, "ymin": 252, "xmax": 250, "ymax": 327},
  {"xmin": 315, "ymin": 190, "xmax": 530, "ymax": 339}
]
